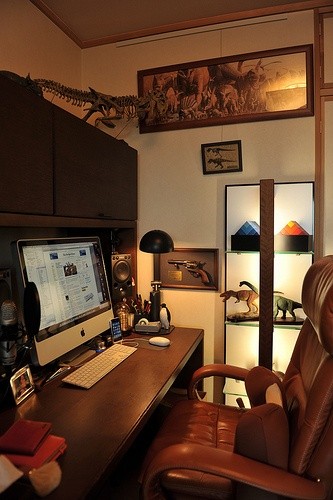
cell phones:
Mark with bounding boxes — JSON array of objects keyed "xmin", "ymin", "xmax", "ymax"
[{"xmin": 110, "ymin": 317, "xmax": 123, "ymax": 344}]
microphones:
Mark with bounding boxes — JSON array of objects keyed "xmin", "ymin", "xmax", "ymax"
[{"xmin": 0, "ymin": 299, "xmax": 18, "ymax": 368}]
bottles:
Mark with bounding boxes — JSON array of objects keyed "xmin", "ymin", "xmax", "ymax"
[{"xmin": 114, "ymin": 301, "xmax": 130, "ymax": 332}]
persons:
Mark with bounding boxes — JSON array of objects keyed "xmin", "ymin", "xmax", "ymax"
[{"xmin": 17, "ymin": 375, "xmax": 31, "ymax": 398}]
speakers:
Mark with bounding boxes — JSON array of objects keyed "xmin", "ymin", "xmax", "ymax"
[{"xmin": 104, "ymin": 253, "xmax": 135, "ymax": 318}]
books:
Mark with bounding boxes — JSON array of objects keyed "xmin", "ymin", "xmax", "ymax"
[{"xmin": 0, "ymin": 419, "xmax": 67, "ymax": 471}]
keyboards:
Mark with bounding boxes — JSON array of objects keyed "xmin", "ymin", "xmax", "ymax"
[{"xmin": 63, "ymin": 344, "xmax": 137, "ymax": 389}]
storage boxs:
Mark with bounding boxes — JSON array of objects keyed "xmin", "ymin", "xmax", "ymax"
[{"xmin": 135, "ymin": 321, "xmax": 162, "ymax": 333}]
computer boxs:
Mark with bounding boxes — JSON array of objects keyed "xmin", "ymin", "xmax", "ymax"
[{"xmin": 155, "ymin": 388, "xmax": 207, "ymax": 422}]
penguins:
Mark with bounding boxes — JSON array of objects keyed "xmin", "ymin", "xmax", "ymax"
[{"xmin": 159, "ymin": 302, "xmax": 172, "ymax": 332}]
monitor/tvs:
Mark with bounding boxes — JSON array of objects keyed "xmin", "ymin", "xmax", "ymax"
[{"xmin": 12, "ymin": 236, "xmax": 115, "ymax": 368}]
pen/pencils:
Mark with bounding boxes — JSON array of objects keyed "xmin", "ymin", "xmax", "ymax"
[{"xmin": 136, "ymin": 294, "xmax": 151, "ymax": 315}]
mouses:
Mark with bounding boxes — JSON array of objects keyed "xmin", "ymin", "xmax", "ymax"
[{"xmin": 149, "ymin": 337, "xmax": 171, "ymax": 346}]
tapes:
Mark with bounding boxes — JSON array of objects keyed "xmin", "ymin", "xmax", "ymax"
[{"xmin": 139, "ymin": 318, "xmax": 149, "ymax": 325}]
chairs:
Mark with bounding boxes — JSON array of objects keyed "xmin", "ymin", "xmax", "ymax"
[{"xmin": 142, "ymin": 254, "xmax": 333, "ymax": 500}]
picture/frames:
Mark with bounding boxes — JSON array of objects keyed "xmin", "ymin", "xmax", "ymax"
[
  {"xmin": 201, "ymin": 139, "xmax": 243, "ymax": 175},
  {"xmin": 137, "ymin": 42, "xmax": 315, "ymax": 134},
  {"xmin": 152, "ymin": 248, "xmax": 220, "ymax": 292},
  {"xmin": 6, "ymin": 362, "xmax": 36, "ymax": 408}
]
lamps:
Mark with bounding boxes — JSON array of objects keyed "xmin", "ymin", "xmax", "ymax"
[{"xmin": 139, "ymin": 229, "xmax": 176, "ymax": 336}]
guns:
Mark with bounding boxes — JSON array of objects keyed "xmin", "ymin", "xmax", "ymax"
[{"xmin": 168, "ymin": 259, "xmax": 210, "ymax": 284}]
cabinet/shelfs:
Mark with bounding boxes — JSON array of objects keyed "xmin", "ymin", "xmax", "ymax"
[
  {"xmin": 0, "ymin": 73, "xmax": 138, "ymax": 229},
  {"xmin": 223, "ymin": 178, "xmax": 315, "ymax": 409}
]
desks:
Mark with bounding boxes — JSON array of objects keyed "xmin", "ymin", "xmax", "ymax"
[{"xmin": 0, "ymin": 325, "xmax": 204, "ymax": 500}]
